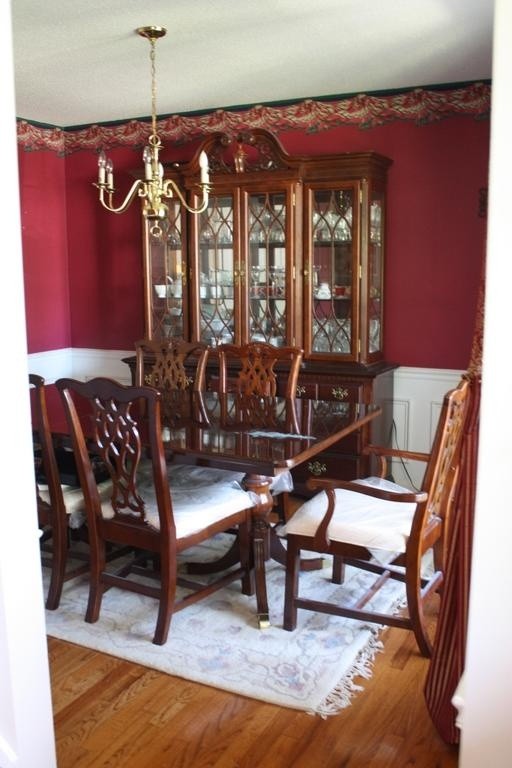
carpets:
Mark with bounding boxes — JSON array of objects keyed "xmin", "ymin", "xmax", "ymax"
[{"xmin": 38, "ymin": 520, "xmax": 440, "ymax": 718}]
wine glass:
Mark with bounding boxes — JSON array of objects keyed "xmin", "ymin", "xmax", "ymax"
[
  {"xmin": 200, "ymin": 264, "xmax": 321, "ymax": 299},
  {"xmin": 203, "ymin": 211, "xmax": 353, "ymax": 243},
  {"xmin": 312, "ymin": 316, "xmax": 379, "ymax": 353}
]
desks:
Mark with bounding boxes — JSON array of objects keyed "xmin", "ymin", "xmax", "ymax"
[{"xmin": 30, "ymin": 379, "xmax": 390, "ymax": 575}]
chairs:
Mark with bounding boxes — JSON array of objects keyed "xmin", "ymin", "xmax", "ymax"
[{"xmin": 275, "ymin": 375, "xmax": 476, "ymax": 657}]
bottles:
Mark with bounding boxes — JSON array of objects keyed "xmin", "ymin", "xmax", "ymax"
[{"xmin": 162, "ymin": 314, "xmax": 172, "ymax": 336}]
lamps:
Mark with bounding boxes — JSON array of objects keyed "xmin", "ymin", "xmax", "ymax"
[{"xmin": 94, "ymin": 28, "xmax": 212, "ymax": 240}]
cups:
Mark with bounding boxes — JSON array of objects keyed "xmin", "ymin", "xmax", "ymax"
[{"xmin": 154, "ymin": 284, "xmax": 166, "ymax": 297}]
